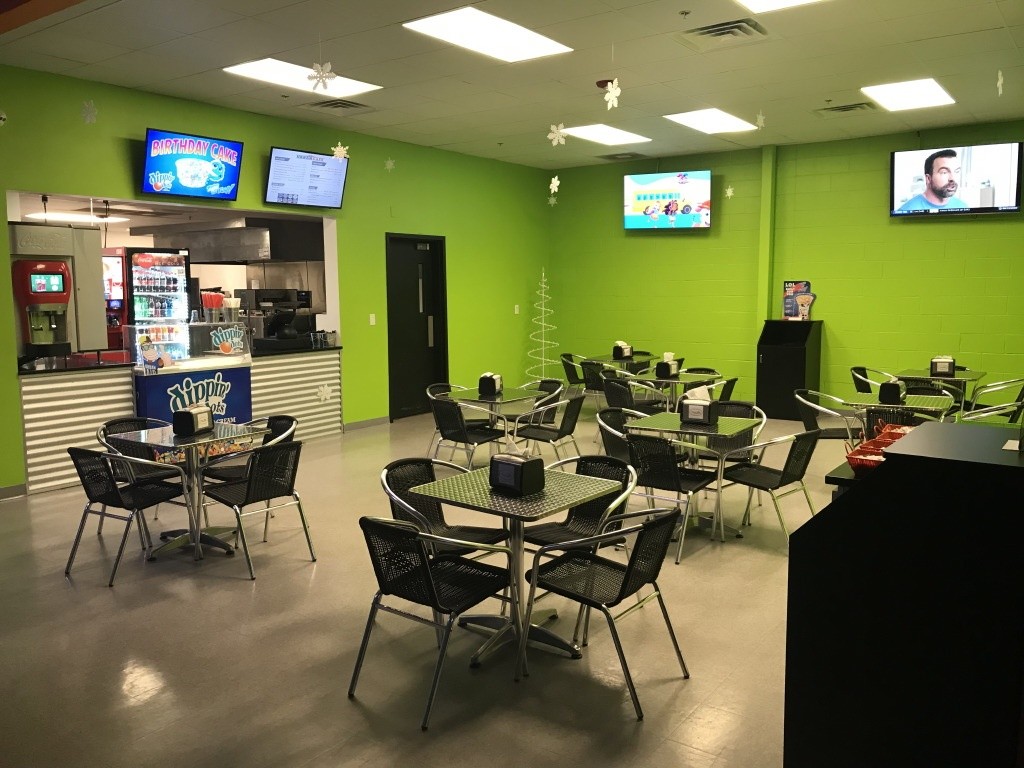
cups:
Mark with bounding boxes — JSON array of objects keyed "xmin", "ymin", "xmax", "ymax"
[{"xmin": 190, "ymin": 310, "xmax": 200, "ymax": 326}]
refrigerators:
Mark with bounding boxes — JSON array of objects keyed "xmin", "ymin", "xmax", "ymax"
[{"xmin": 118, "ymin": 243, "xmax": 192, "ymax": 365}]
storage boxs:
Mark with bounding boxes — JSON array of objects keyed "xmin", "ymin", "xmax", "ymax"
[
  {"xmin": 872, "ymin": 426, "xmax": 906, "ymax": 442},
  {"xmin": 859, "ymin": 431, "xmax": 896, "ymax": 451},
  {"xmin": 845, "ymin": 441, "xmax": 887, "ymax": 472},
  {"xmin": 878, "ymin": 417, "xmax": 914, "ymax": 434}
]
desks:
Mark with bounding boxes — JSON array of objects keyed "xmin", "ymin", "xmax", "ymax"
[
  {"xmin": 106, "ymin": 420, "xmax": 274, "ymax": 562},
  {"xmin": 622, "ymin": 411, "xmax": 761, "ymax": 542},
  {"xmin": 841, "ymin": 392, "xmax": 954, "ymax": 425},
  {"xmin": 435, "ymin": 386, "xmax": 551, "ymax": 457},
  {"xmin": 892, "ymin": 369, "xmax": 988, "ymax": 390},
  {"xmin": 619, "ymin": 371, "xmax": 723, "ymax": 406},
  {"xmin": 406, "ymin": 467, "xmax": 623, "ymax": 672},
  {"xmin": 584, "ymin": 353, "xmax": 661, "ymax": 392}
]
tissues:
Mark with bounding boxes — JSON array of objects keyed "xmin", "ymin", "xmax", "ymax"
[
  {"xmin": 656, "ymin": 353, "xmax": 680, "ymax": 378},
  {"xmin": 928, "ymin": 356, "xmax": 956, "ymax": 376},
  {"xmin": 879, "ymin": 372, "xmax": 907, "ymax": 403},
  {"xmin": 173, "ymin": 401, "xmax": 216, "ymax": 436},
  {"xmin": 487, "ymin": 436, "xmax": 546, "ymax": 495},
  {"xmin": 680, "ymin": 386, "xmax": 718, "ymax": 425},
  {"xmin": 613, "ymin": 341, "xmax": 634, "ymax": 360},
  {"xmin": 479, "ymin": 371, "xmax": 504, "ymax": 395}
]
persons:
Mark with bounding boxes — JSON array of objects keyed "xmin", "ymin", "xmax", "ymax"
[{"xmin": 894, "ymin": 150, "xmax": 971, "ymax": 212}]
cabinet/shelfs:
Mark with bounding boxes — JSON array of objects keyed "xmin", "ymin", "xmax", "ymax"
[{"xmin": 824, "ymin": 460, "xmax": 861, "ymax": 502}]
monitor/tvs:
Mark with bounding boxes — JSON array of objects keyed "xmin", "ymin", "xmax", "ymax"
[
  {"xmin": 265, "ymin": 146, "xmax": 349, "ymax": 209},
  {"xmin": 890, "ymin": 141, "xmax": 1023, "ymax": 217},
  {"xmin": 142, "ymin": 128, "xmax": 244, "ymax": 202},
  {"xmin": 624, "ymin": 169, "xmax": 711, "ymax": 229}
]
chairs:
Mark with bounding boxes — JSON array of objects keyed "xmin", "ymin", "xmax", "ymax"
[
  {"xmin": 674, "ymin": 378, "xmax": 739, "ymax": 412},
  {"xmin": 709, "ymin": 428, "xmax": 822, "ymax": 549},
  {"xmin": 423, "ymin": 384, "xmax": 469, "ymax": 461},
  {"xmin": 580, "ymin": 361, "xmax": 630, "ymax": 413},
  {"xmin": 849, "ymin": 366, "xmax": 898, "ymax": 391},
  {"xmin": 661, "ymin": 366, "xmax": 723, "ymax": 401},
  {"xmin": 615, "ymin": 433, "xmax": 727, "ymax": 565},
  {"xmin": 854, "ymin": 407, "xmax": 941, "ymax": 441},
  {"xmin": 347, "ymin": 516, "xmax": 532, "ymax": 732},
  {"xmin": 203, "ymin": 414, "xmax": 300, "ymax": 543},
  {"xmin": 65, "ymin": 447, "xmax": 205, "ymax": 588},
  {"xmin": 626, "ymin": 351, "xmax": 656, "ymax": 400},
  {"xmin": 678, "ymin": 400, "xmax": 767, "ymax": 528},
  {"xmin": 513, "ymin": 507, "xmax": 691, "ymax": 722},
  {"xmin": 602, "ymin": 379, "xmax": 669, "ymax": 415},
  {"xmin": 631, "ymin": 357, "xmax": 685, "ymax": 406},
  {"xmin": 497, "ymin": 453, "xmax": 638, "ymax": 643},
  {"xmin": 596, "ymin": 408, "xmax": 691, "ymax": 524},
  {"xmin": 505, "ymin": 395, "xmax": 585, "ymax": 472},
  {"xmin": 794, "ymin": 388, "xmax": 867, "ymax": 450},
  {"xmin": 194, "ymin": 440, "xmax": 318, "ymax": 581},
  {"xmin": 560, "ymin": 353, "xmax": 605, "ymax": 412},
  {"xmin": 963, "ymin": 378, "xmax": 1024, "ymax": 424},
  {"xmin": 506, "ymin": 379, "xmax": 564, "ymax": 455},
  {"xmin": 599, "ymin": 368, "xmax": 657, "ymax": 402},
  {"xmin": 925, "ymin": 365, "xmax": 979, "ymax": 417},
  {"xmin": 430, "ymin": 396, "xmax": 508, "ymax": 472},
  {"xmin": 95, "ymin": 416, "xmax": 210, "ymax": 552},
  {"xmin": 378, "ymin": 457, "xmax": 514, "ymax": 652}
]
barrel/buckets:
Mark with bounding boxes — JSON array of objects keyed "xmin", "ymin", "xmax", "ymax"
[
  {"xmin": 223, "ymin": 307, "xmax": 240, "ymax": 322},
  {"xmin": 203, "ymin": 306, "xmax": 222, "ymax": 324}
]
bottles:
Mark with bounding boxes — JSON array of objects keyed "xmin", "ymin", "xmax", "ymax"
[{"xmin": 133, "ymin": 264, "xmax": 187, "ymax": 360}]
